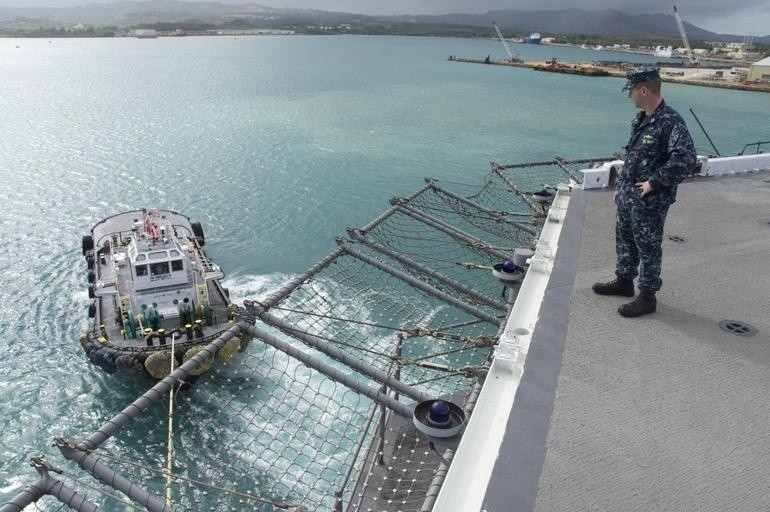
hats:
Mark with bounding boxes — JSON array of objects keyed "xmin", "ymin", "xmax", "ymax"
[{"xmin": 620, "ymin": 67, "xmax": 660, "ymax": 93}]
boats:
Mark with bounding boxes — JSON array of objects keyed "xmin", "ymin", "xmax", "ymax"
[{"xmin": 82, "ymin": 207, "xmax": 255, "ymax": 390}]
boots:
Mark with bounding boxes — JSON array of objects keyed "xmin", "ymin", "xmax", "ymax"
[
  {"xmin": 618, "ymin": 292, "xmax": 656, "ymax": 317},
  {"xmin": 591, "ymin": 278, "xmax": 634, "ymax": 297}
]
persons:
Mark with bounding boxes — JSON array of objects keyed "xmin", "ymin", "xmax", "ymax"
[{"xmin": 592, "ymin": 67, "xmax": 696, "ymax": 317}]
